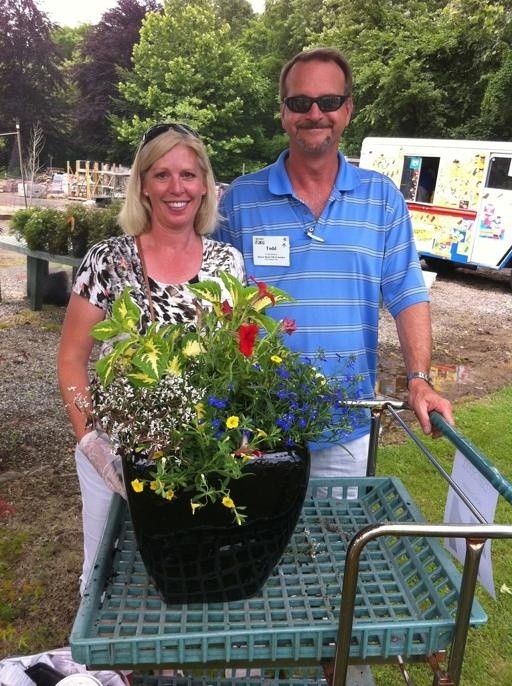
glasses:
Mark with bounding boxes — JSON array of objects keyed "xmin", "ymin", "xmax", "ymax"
[
  {"xmin": 140, "ymin": 124, "xmax": 198, "ymax": 149},
  {"xmin": 282, "ymin": 95, "xmax": 350, "ymax": 112}
]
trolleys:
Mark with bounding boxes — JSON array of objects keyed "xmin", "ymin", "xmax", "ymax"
[{"xmin": 66, "ymin": 397, "xmax": 512, "ymax": 686}]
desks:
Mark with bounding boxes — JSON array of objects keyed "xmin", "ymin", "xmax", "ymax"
[{"xmin": 0, "ymin": 235, "xmax": 105, "ymax": 310}]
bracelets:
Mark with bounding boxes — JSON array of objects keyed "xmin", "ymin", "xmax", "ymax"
[{"xmin": 406, "ymin": 371, "xmax": 436, "ymax": 391}]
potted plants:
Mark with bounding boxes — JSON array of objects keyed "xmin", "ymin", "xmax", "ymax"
[
  {"xmin": 23, "ymin": 208, "xmax": 70, "ymax": 255},
  {"xmin": 65, "ymin": 204, "xmax": 92, "ymax": 259},
  {"xmin": 8, "ymin": 205, "xmax": 47, "ymax": 251},
  {"xmin": 61, "ymin": 272, "xmax": 373, "ymax": 605}
]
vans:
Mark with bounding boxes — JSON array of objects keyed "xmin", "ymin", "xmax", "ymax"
[{"xmin": 358, "ymin": 135, "xmax": 512, "ymax": 277}]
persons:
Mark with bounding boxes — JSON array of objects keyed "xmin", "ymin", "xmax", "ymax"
[
  {"xmin": 204, "ymin": 48, "xmax": 455, "ymax": 502},
  {"xmin": 59, "ymin": 123, "xmax": 244, "ymax": 603}
]
